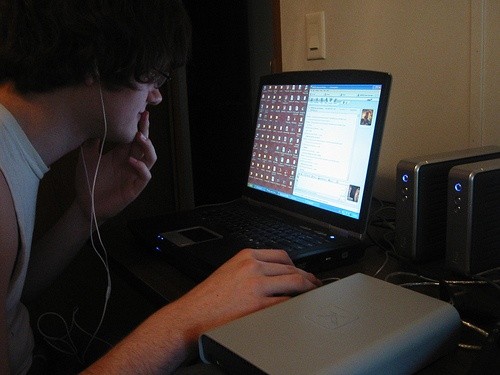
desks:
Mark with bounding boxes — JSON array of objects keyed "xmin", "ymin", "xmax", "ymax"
[{"xmin": 106, "ymin": 200, "xmax": 500, "ymax": 375}]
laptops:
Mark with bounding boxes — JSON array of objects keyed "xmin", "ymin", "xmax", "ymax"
[{"xmin": 127, "ymin": 69, "xmax": 392, "ymax": 285}]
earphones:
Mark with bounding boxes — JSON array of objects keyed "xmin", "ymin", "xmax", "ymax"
[{"xmin": 93, "ymin": 59, "xmax": 100, "ymax": 81}]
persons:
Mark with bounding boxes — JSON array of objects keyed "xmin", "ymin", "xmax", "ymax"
[{"xmin": 0, "ymin": 0, "xmax": 325, "ymax": 375}]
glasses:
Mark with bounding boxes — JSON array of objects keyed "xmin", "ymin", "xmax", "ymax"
[{"xmin": 143, "ymin": 66, "xmax": 174, "ymax": 85}]
